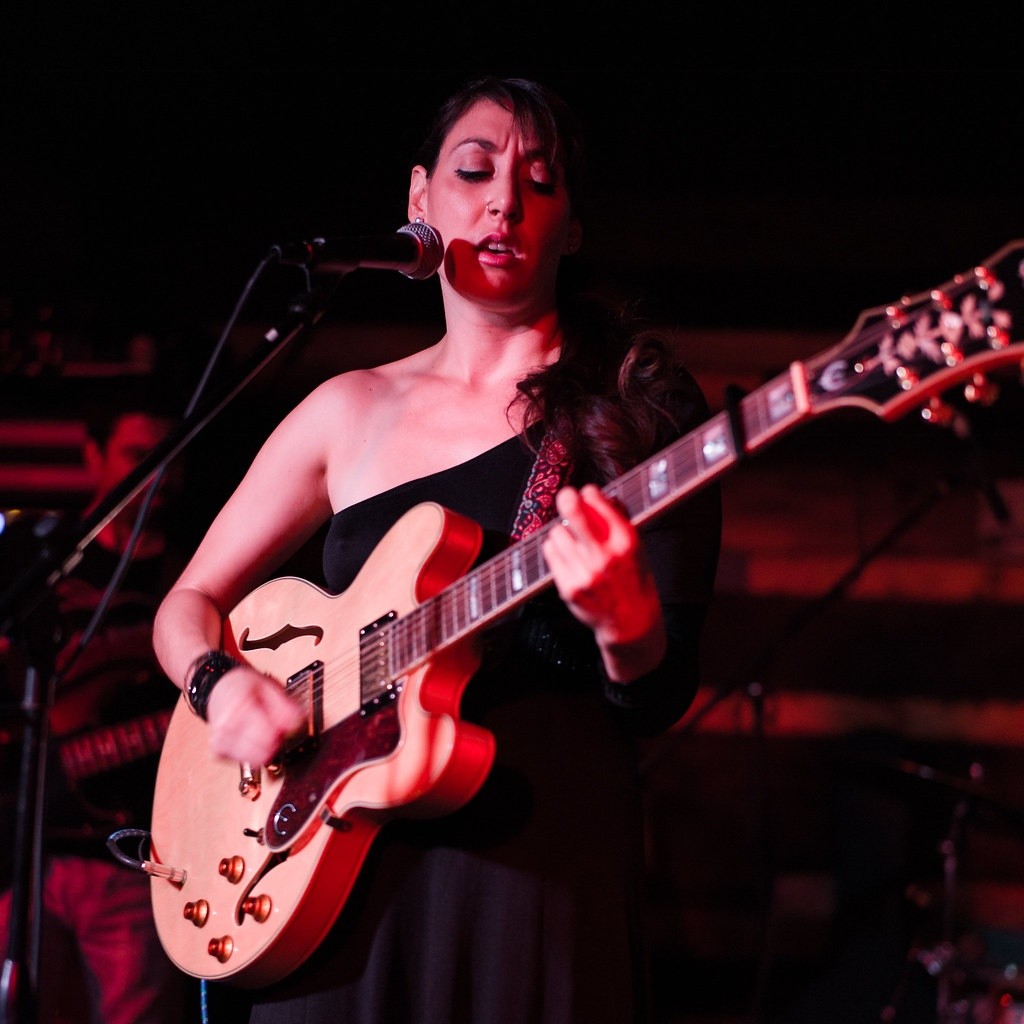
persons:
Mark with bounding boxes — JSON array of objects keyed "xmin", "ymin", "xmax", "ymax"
[
  {"xmin": 150, "ymin": 80, "xmax": 723, "ymax": 1024},
  {"xmin": 31, "ymin": 385, "xmax": 197, "ymax": 1024}
]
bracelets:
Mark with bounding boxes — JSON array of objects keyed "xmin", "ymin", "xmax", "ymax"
[{"xmin": 183, "ymin": 649, "xmax": 238, "ymax": 721}]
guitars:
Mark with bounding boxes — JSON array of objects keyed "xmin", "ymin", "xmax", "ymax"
[
  {"xmin": 147, "ymin": 229, "xmax": 1023, "ymax": 996},
  {"xmin": 0, "ymin": 705, "xmax": 175, "ymax": 901}
]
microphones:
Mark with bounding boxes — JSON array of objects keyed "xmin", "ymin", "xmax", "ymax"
[
  {"xmin": 271, "ymin": 222, "xmax": 444, "ymax": 280},
  {"xmin": 952, "ymin": 414, "xmax": 1010, "ymax": 522}
]
